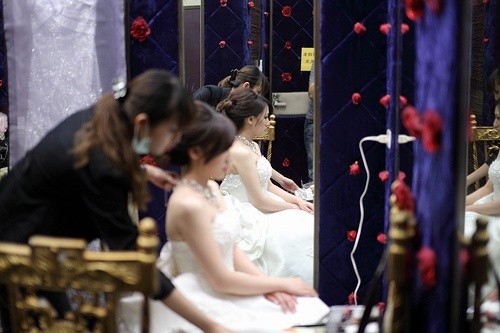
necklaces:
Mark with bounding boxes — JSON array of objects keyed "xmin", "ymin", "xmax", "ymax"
[
  {"xmin": 173, "ymin": 178, "xmax": 218, "ymax": 205},
  {"xmin": 235, "ymin": 134, "xmax": 261, "ymax": 157}
]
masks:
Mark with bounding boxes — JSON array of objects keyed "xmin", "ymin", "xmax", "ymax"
[{"xmin": 129, "ymin": 122, "xmax": 152, "ymax": 154}]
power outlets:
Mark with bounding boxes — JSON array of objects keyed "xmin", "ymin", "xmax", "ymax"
[{"xmin": 385, "ymin": 129, "xmax": 392, "ymax": 149}]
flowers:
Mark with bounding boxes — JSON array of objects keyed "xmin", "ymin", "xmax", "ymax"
[{"xmin": 130, "ymin": 18, "xmax": 150, "ymax": 42}]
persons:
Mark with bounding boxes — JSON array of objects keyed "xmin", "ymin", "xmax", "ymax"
[
  {"xmin": 166, "ymin": 100, "xmax": 331, "ymax": 333},
  {"xmin": 466, "ymin": 68, "xmax": 500, "ymax": 186},
  {"xmin": 215, "ymin": 87, "xmax": 314, "ymax": 289},
  {"xmin": 0, "ymin": 68, "xmax": 233, "ymax": 333},
  {"xmin": 304, "ymin": 62, "xmax": 315, "ymax": 182},
  {"xmin": 191, "ymin": 65, "xmax": 300, "ymax": 194},
  {"xmin": 464, "ymin": 99, "xmax": 500, "ymax": 261}
]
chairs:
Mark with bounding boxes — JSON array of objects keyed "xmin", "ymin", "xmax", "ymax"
[
  {"xmin": 0, "ymin": 216, "xmax": 159, "ymax": 333},
  {"xmin": 472, "ymin": 116, "xmax": 500, "ymax": 191}
]
